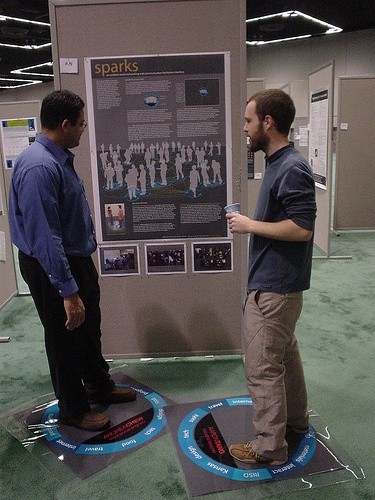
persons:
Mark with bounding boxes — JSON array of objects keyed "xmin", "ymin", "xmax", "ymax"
[
  {"xmin": 107, "ymin": 206, "xmax": 114, "ymax": 224},
  {"xmin": 194, "ymin": 245, "xmax": 231, "ymax": 271},
  {"xmin": 8, "ymin": 89, "xmax": 138, "ymax": 432},
  {"xmin": 117, "ymin": 205, "xmax": 124, "ymax": 228},
  {"xmin": 102, "ymin": 249, "xmax": 136, "ymax": 274},
  {"xmin": 149, "ymin": 248, "xmax": 184, "ymax": 267},
  {"xmin": 225, "ymin": 88, "xmax": 318, "ymax": 464}
]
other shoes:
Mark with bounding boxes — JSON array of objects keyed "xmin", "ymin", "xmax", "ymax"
[
  {"xmin": 85, "ymin": 387, "xmax": 137, "ymax": 403},
  {"xmin": 60, "ymin": 409, "xmax": 110, "ymax": 431}
]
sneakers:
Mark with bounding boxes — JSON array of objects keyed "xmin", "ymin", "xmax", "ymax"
[
  {"xmin": 290, "ymin": 426, "xmax": 310, "ymax": 434},
  {"xmin": 230, "ymin": 443, "xmax": 257, "ymax": 461}
]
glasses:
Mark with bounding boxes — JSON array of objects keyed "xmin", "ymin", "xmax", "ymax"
[{"xmin": 59, "ymin": 120, "xmax": 88, "ymax": 128}]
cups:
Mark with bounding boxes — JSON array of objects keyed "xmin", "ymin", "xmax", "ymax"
[{"xmin": 224, "ymin": 203, "xmax": 241, "ymax": 220}]
